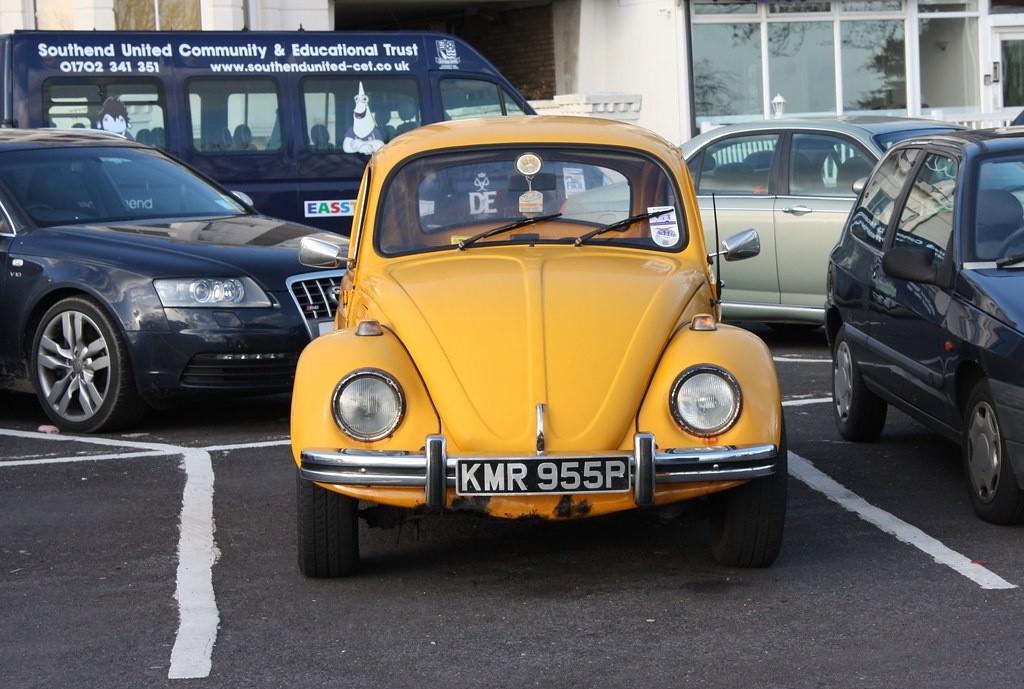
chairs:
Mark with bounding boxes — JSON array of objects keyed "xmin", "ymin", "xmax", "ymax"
[
  {"xmin": 65, "ymin": 96, "xmax": 421, "ymax": 154},
  {"xmin": 975, "ymin": 188, "xmax": 1023, "ymax": 259},
  {"xmin": 689, "ymin": 145, "xmax": 877, "ymax": 201}
]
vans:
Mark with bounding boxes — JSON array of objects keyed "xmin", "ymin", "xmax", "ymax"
[{"xmin": 3, "ymin": 29, "xmax": 540, "ymax": 239}]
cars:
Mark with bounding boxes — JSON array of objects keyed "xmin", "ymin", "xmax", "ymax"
[
  {"xmin": 285, "ymin": 112, "xmax": 789, "ymax": 580},
  {"xmin": 1, "ymin": 125, "xmax": 348, "ymax": 442},
  {"xmin": 553, "ymin": 117, "xmax": 965, "ymax": 326},
  {"xmin": 824, "ymin": 125, "xmax": 1022, "ymax": 528}
]
